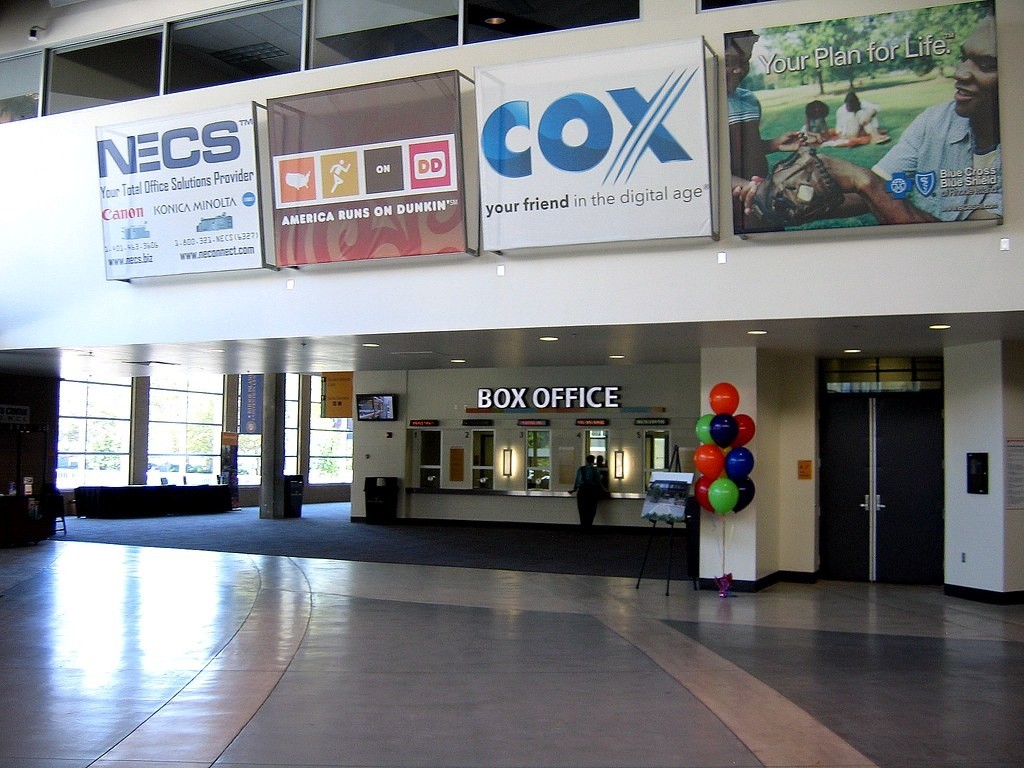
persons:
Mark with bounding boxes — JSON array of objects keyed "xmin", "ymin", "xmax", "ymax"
[
  {"xmin": 805, "ymin": 100, "xmax": 830, "ymax": 143},
  {"xmin": 836, "ymin": 92, "xmax": 890, "ymax": 144},
  {"xmin": 724, "ymin": 30, "xmax": 809, "ymax": 191},
  {"xmin": 228, "ymin": 469, "xmax": 240, "ymax": 508},
  {"xmin": 734, "ymin": 11, "xmax": 1004, "ymax": 223},
  {"xmin": 567, "ymin": 455, "xmax": 610, "ymax": 530}
]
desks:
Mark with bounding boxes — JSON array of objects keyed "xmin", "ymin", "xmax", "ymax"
[{"xmin": 74, "ymin": 485, "xmax": 232, "ymax": 518}]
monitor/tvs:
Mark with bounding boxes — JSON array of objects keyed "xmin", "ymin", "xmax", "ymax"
[{"xmin": 356, "ymin": 394, "xmax": 398, "ymax": 421}]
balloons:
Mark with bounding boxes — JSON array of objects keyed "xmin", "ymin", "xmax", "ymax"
[
  {"xmin": 708, "ymin": 478, "xmax": 739, "ymax": 515},
  {"xmin": 731, "ymin": 477, "xmax": 755, "ymax": 513},
  {"xmin": 720, "ymin": 446, "xmax": 755, "ymax": 478},
  {"xmin": 696, "ymin": 414, "xmax": 716, "ymax": 445},
  {"xmin": 731, "ymin": 414, "xmax": 755, "ymax": 449},
  {"xmin": 695, "ymin": 477, "xmax": 718, "ymax": 514},
  {"xmin": 710, "ymin": 413, "xmax": 739, "ymax": 449},
  {"xmin": 694, "ymin": 444, "xmax": 724, "ymax": 481},
  {"xmin": 710, "ymin": 382, "xmax": 739, "ymax": 415}
]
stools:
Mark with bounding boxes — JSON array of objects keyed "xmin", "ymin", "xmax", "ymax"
[{"xmin": 53, "ymin": 514, "xmax": 67, "ymax": 534}]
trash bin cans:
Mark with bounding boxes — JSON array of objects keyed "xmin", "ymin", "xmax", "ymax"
[
  {"xmin": 363, "ymin": 476, "xmax": 399, "ymax": 523},
  {"xmin": 284, "ymin": 473, "xmax": 304, "ymax": 519}
]
